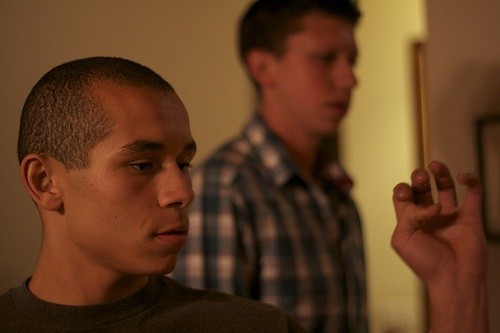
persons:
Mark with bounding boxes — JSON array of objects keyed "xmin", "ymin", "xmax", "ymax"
[
  {"xmin": 0, "ymin": 57, "xmax": 489, "ymax": 333},
  {"xmin": 166, "ymin": 0, "xmax": 370, "ymax": 333}
]
